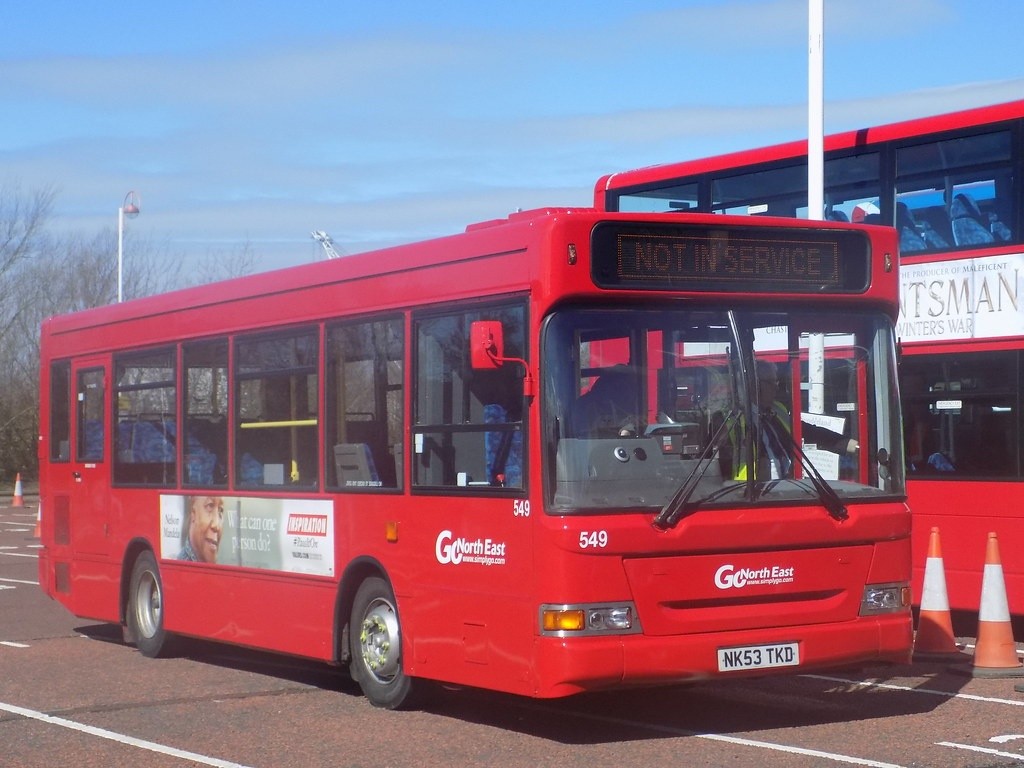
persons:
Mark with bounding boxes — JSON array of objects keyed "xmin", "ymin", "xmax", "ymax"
[
  {"xmin": 707, "ymin": 359, "xmax": 860, "ymax": 482},
  {"xmin": 176, "ymin": 496, "xmax": 225, "ymax": 564}
]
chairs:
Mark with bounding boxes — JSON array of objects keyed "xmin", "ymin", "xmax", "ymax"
[
  {"xmin": 84, "ymin": 411, "xmax": 381, "ymax": 487},
  {"xmin": 591, "ymin": 363, "xmax": 678, "ymax": 436},
  {"xmin": 829, "ymin": 194, "xmax": 1011, "ymax": 253},
  {"xmin": 928, "ymin": 452, "xmax": 959, "ymax": 473},
  {"xmin": 485, "ymin": 404, "xmax": 524, "ymax": 489}
]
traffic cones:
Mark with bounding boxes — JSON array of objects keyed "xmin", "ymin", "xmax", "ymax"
[
  {"xmin": 906, "ymin": 525, "xmax": 975, "ymax": 665},
  {"xmin": 11, "ymin": 472, "xmax": 28, "ymax": 507},
  {"xmin": 947, "ymin": 533, "xmax": 1022, "ymax": 678},
  {"xmin": 32, "ymin": 497, "xmax": 41, "ymax": 539}
]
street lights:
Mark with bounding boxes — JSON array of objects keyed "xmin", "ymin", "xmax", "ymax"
[{"xmin": 117, "ymin": 191, "xmax": 142, "ymax": 305}]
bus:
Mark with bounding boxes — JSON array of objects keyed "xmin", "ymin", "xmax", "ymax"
[
  {"xmin": 591, "ymin": 98, "xmax": 1024, "ymax": 630},
  {"xmin": 37, "ymin": 207, "xmax": 935, "ymax": 714}
]
